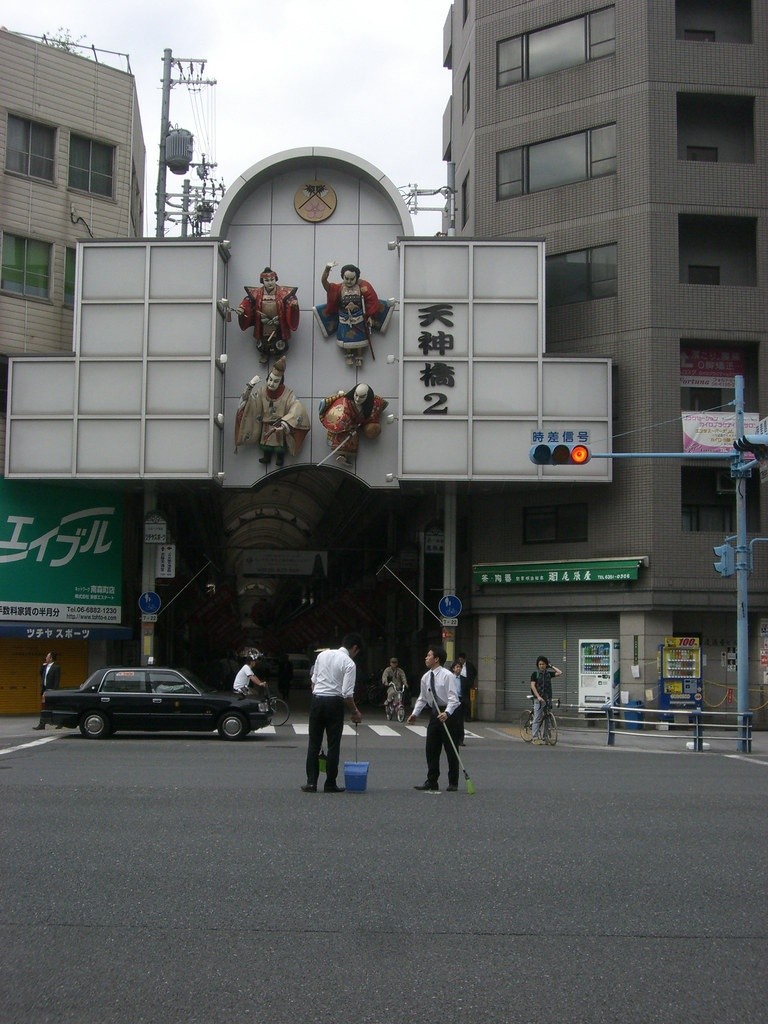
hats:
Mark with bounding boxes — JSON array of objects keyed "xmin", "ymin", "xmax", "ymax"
[{"xmin": 390, "ymin": 658, "xmax": 398, "ymax": 663}]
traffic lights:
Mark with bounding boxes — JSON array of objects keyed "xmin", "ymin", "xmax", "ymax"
[
  {"xmin": 712, "ymin": 545, "xmax": 736, "ymax": 577},
  {"xmin": 529, "ymin": 443, "xmax": 592, "ymax": 465},
  {"xmin": 732, "ymin": 435, "xmax": 768, "ymax": 462}
]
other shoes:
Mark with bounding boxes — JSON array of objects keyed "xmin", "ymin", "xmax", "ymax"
[
  {"xmin": 301, "ymin": 784, "xmax": 318, "ymax": 792},
  {"xmin": 324, "ymin": 784, "xmax": 346, "ymax": 793},
  {"xmin": 384, "ymin": 700, "xmax": 389, "ymax": 706},
  {"xmin": 465, "ymin": 716, "xmax": 471, "ymax": 722},
  {"xmin": 459, "ymin": 744, "xmax": 466, "ymax": 746},
  {"xmin": 446, "ymin": 784, "xmax": 458, "ymax": 791},
  {"xmin": 32, "ymin": 723, "xmax": 46, "ymax": 730},
  {"xmin": 533, "ymin": 739, "xmax": 542, "ymax": 745},
  {"xmin": 55, "ymin": 725, "xmax": 63, "ymax": 729},
  {"xmin": 414, "ymin": 780, "xmax": 440, "ymax": 790}
]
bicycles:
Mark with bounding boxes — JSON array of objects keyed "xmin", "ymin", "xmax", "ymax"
[
  {"xmin": 353, "ymin": 681, "xmax": 387, "ymax": 709},
  {"xmin": 385, "ymin": 683, "xmax": 410, "ymax": 722},
  {"xmin": 519, "ymin": 697, "xmax": 561, "ymax": 745},
  {"xmin": 241, "ymin": 685, "xmax": 291, "ymax": 727}
]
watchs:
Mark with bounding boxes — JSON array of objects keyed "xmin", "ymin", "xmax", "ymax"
[{"xmin": 550, "ymin": 664, "xmax": 553, "ymax": 668}]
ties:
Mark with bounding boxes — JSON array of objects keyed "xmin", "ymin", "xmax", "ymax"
[{"xmin": 431, "ymin": 672, "xmax": 437, "ymax": 701}]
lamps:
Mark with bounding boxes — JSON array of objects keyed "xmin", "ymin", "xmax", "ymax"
[
  {"xmin": 385, "ymin": 473, "xmax": 398, "ymax": 482},
  {"xmin": 388, "ymin": 240, "xmax": 400, "ymax": 250},
  {"xmin": 218, "ymin": 298, "xmax": 229, "ymax": 307},
  {"xmin": 214, "ymin": 413, "xmax": 224, "ymax": 424},
  {"xmin": 386, "ymin": 354, "xmax": 401, "ymax": 364},
  {"xmin": 218, "ymin": 353, "xmax": 227, "ymax": 364},
  {"xmin": 386, "ymin": 414, "xmax": 399, "ymax": 424},
  {"xmin": 220, "ymin": 240, "xmax": 231, "ymax": 249},
  {"xmin": 215, "ymin": 471, "xmax": 226, "ymax": 481},
  {"xmin": 387, "ymin": 297, "xmax": 400, "ymax": 305}
]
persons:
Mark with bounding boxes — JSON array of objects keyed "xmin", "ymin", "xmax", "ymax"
[
  {"xmin": 407, "ymin": 648, "xmax": 465, "ymax": 791},
  {"xmin": 301, "ymin": 633, "xmax": 363, "ymax": 792},
  {"xmin": 233, "ymin": 657, "xmax": 268, "ymax": 700},
  {"xmin": 31, "ymin": 651, "xmax": 62, "ymax": 730},
  {"xmin": 275, "ymin": 653, "xmax": 478, "ymax": 745},
  {"xmin": 529, "ymin": 656, "xmax": 564, "ymax": 745},
  {"xmin": 234, "ymin": 261, "xmax": 387, "ymax": 468}
]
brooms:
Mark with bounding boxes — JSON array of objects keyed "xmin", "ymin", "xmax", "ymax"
[{"xmin": 428, "ymin": 688, "xmax": 475, "ymax": 794}]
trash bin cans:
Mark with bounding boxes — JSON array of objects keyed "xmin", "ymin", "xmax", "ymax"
[{"xmin": 624, "ymin": 700, "xmax": 644, "ymax": 730}]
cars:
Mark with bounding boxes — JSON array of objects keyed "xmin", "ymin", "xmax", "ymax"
[{"xmin": 40, "ymin": 667, "xmax": 273, "ymax": 741}]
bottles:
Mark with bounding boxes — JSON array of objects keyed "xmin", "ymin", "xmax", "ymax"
[
  {"xmin": 584, "ymin": 645, "xmax": 609, "ymax": 674},
  {"xmin": 668, "ymin": 649, "xmax": 694, "ymax": 677}
]
